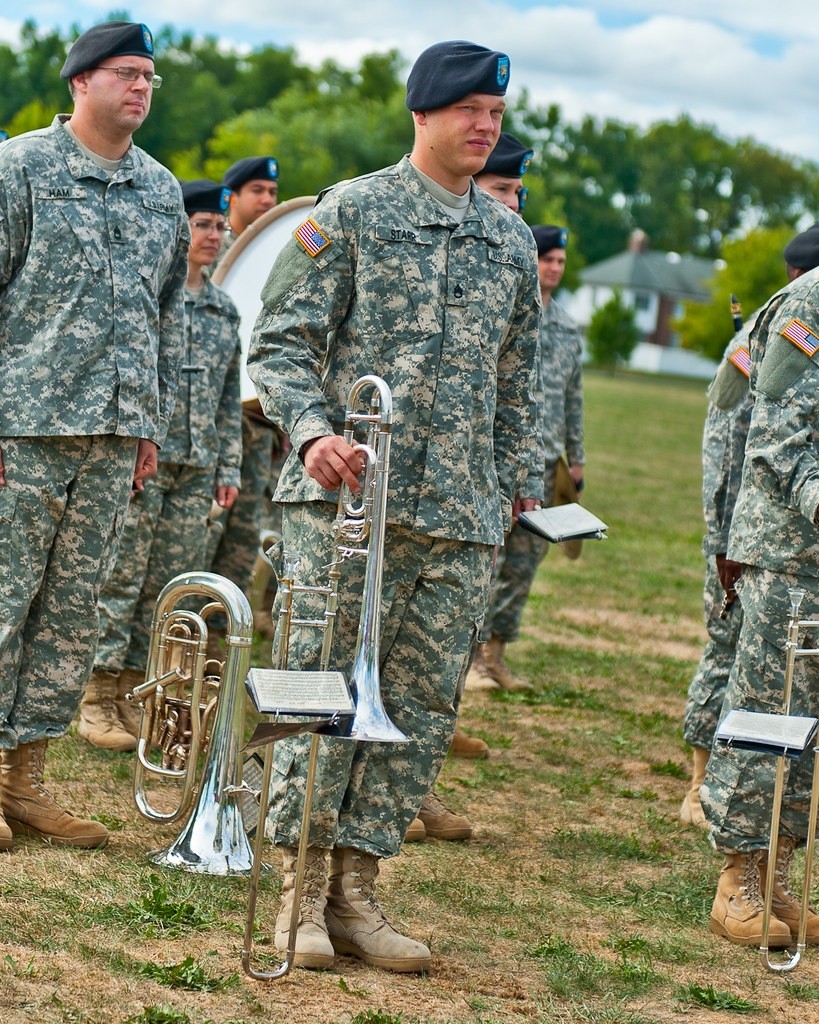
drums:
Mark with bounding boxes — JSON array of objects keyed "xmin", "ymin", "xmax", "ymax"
[{"xmin": 209, "ymin": 195, "xmax": 322, "ymax": 428}]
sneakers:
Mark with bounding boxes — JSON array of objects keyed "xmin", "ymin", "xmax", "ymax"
[
  {"xmin": 449, "ymin": 725, "xmax": 488, "ymax": 760},
  {"xmin": 418, "ymin": 790, "xmax": 472, "ymax": 840},
  {"xmin": 404, "ymin": 816, "xmax": 426, "ymax": 843}
]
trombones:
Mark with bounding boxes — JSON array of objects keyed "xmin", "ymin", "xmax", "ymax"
[
  {"xmin": 759, "ymin": 587, "xmax": 819, "ymax": 974},
  {"xmin": 243, "ymin": 372, "xmax": 411, "ymax": 982}
]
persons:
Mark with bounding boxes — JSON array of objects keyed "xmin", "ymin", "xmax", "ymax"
[
  {"xmin": 683, "ymin": 226, "xmax": 819, "ymax": 947},
  {"xmin": 243, "ymin": 41, "xmax": 546, "ymax": 973},
  {"xmin": 77, "ymin": 129, "xmax": 546, "ymax": 844},
  {"xmin": 467, "ymin": 225, "xmax": 586, "ymax": 692},
  {"xmin": 0, "ymin": 21, "xmax": 191, "ymax": 851}
]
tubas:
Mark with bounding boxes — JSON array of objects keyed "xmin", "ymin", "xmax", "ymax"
[{"xmin": 126, "ymin": 568, "xmax": 258, "ymax": 876}]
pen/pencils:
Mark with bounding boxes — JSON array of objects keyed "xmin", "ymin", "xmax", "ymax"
[{"xmin": 540, "ymin": 509, "xmax": 564, "ymax": 536}]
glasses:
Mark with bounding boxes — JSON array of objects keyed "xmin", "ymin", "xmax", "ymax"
[
  {"xmin": 186, "ymin": 218, "xmax": 232, "ymax": 239},
  {"xmin": 90, "ymin": 59, "xmax": 161, "ymax": 91}
]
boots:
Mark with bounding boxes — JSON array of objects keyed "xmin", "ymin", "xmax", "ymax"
[
  {"xmin": 712, "ymin": 844, "xmax": 792, "ymax": 947},
  {"xmin": 482, "ymin": 638, "xmax": 534, "ymax": 693},
  {"xmin": 463, "ymin": 640, "xmax": 502, "ymax": 692},
  {"xmin": 323, "ymin": 848, "xmax": 432, "ymax": 971},
  {"xmin": 118, "ymin": 667, "xmax": 162, "ymax": 751},
  {"xmin": 76, "ymin": 669, "xmax": 139, "ymax": 753},
  {"xmin": 678, "ymin": 746, "xmax": 710, "ymax": 831},
  {"xmin": 2, "ymin": 738, "xmax": 110, "ymax": 847},
  {"xmin": 274, "ymin": 847, "xmax": 335, "ymax": 968},
  {"xmin": 0, "ymin": 808, "xmax": 12, "ymax": 853},
  {"xmin": 759, "ymin": 835, "xmax": 819, "ymax": 947}
]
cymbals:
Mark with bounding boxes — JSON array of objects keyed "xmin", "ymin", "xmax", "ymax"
[{"xmin": 554, "ymin": 456, "xmax": 583, "ymax": 560}]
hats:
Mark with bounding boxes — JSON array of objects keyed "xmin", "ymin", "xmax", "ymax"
[
  {"xmin": 518, "ymin": 188, "xmax": 528, "ymax": 213},
  {"xmin": 527, "ymin": 223, "xmax": 568, "ymax": 259},
  {"xmin": 406, "ymin": 39, "xmax": 511, "ymax": 113},
  {"xmin": 472, "ymin": 132, "xmax": 534, "ymax": 179},
  {"xmin": 180, "ymin": 179, "xmax": 231, "ymax": 214},
  {"xmin": 219, "ymin": 154, "xmax": 281, "ymax": 187},
  {"xmin": 60, "ymin": 21, "xmax": 155, "ymax": 81},
  {"xmin": 785, "ymin": 220, "xmax": 819, "ymax": 270}
]
